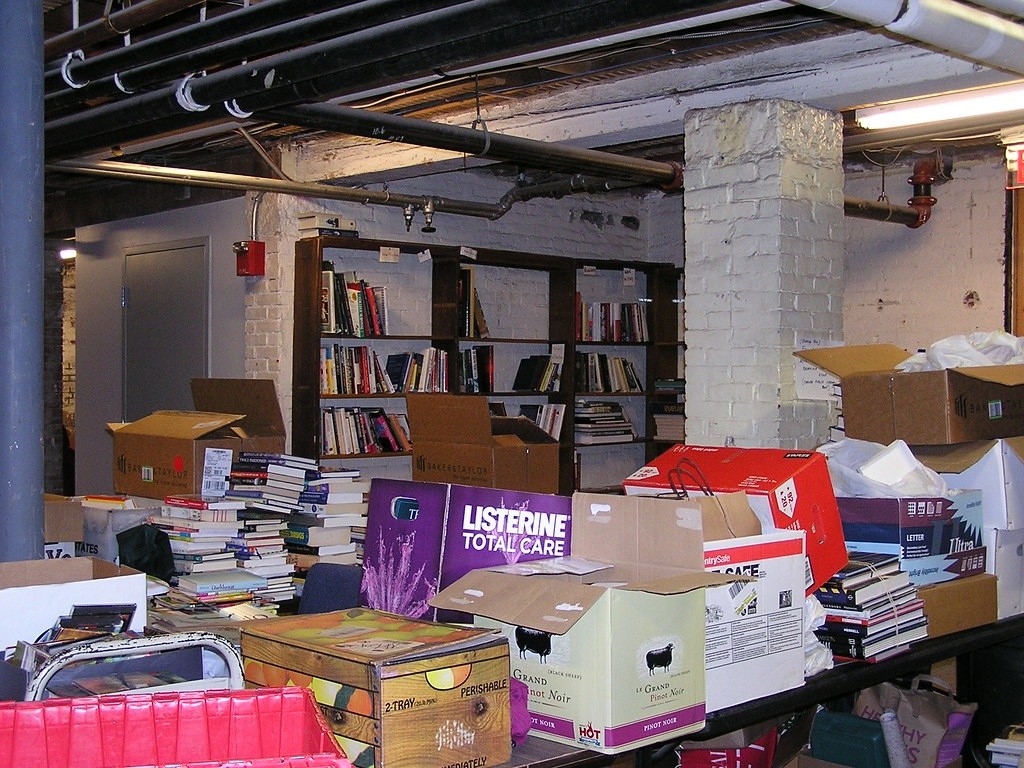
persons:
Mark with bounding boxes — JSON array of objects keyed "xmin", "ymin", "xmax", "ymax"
[{"xmin": 62, "ymin": 425, "xmax": 75, "ymax": 496}]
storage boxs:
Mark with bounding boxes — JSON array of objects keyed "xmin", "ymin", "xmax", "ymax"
[
  {"xmin": 624, "ymin": 443, "xmax": 851, "ymax": 599},
  {"xmin": 833, "ymin": 487, "xmax": 987, "ymax": 588},
  {"xmin": 0, "ymin": 684, "xmax": 354, "ymax": 768},
  {"xmin": 904, "ymin": 435, "xmax": 1024, "ymax": 530},
  {"xmin": 43, "ymin": 492, "xmax": 85, "ymax": 542},
  {"xmin": 358, "ymin": 476, "xmax": 575, "ymax": 625},
  {"xmin": 798, "ymin": 749, "xmax": 851, "ymax": 768},
  {"xmin": 44, "ymin": 542, "xmax": 75, "ymax": 559},
  {"xmin": 102, "ymin": 375, "xmax": 289, "ymax": 504},
  {"xmin": 77, "ymin": 493, "xmax": 166, "ymax": 564},
  {"xmin": 683, "ymin": 491, "xmax": 808, "ymax": 713},
  {"xmin": 237, "ymin": 605, "xmax": 514, "ymax": 768},
  {"xmin": 791, "ymin": 341, "xmax": 1024, "ymax": 446},
  {"xmin": 426, "ymin": 491, "xmax": 761, "ymax": 757},
  {"xmin": 0, "ymin": 555, "xmax": 149, "ymax": 662},
  {"xmin": 978, "ymin": 527, "xmax": 1024, "ymax": 620},
  {"xmin": 912, "ymin": 573, "xmax": 1000, "ymax": 639}
]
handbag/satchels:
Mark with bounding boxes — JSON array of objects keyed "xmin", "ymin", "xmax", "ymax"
[{"xmin": 854, "ymin": 673, "xmax": 979, "ymax": 768}]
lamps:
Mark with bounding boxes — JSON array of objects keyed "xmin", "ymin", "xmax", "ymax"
[{"xmin": 853, "ymin": 80, "xmax": 1024, "ymax": 132}]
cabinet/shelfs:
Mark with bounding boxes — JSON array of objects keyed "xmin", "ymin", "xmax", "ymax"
[{"xmin": 290, "ymin": 235, "xmax": 685, "ymax": 494}]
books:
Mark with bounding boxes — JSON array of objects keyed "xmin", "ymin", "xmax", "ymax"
[
  {"xmin": 81, "ymin": 496, "xmax": 136, "ymax": 510},
  {"xmin": 650, "ymin": 403, "xmax": 685, "ymax": 440},
  {"xmin": 5, "ymin": 603, "xmax": 187, "ymax": 698},
  {"xmin": 575, "ymin": 399, "xmax": 639, "ymax": 445},
  {"xmin": 575, "ymin": 291, "xmax": 649, "ymax": 342},
  {"xmin": 488, "ymin": 401, "xmax": 507, "ymax": 416},
  {"xmin": 519, "ymin": 404, "xmax": 566, "ymax": 441},
  {"xmin": 460, "ymin": 267, "xmax": 490, "ymax": 340},
  {"xmin": 320, "ymin": 260, "xmax": 389, "ymax": 337},
  {"xmin": 575, "ymin": 350, "xmax": 644, "ymax": 393},
  {"xmin": 460, "ymin": 346, "xmax": 494, "ymax": 393},
  {"xmin": 812, "ymin": 552, "xmax": 929, "ymax": 664},
  {"xmin": 321, "ymin": 406, "xmax": 413, "ymax": 454},
  {"xmin": 986, "ymin": 722, "xmax": 1024, "ymax": 768},
  {"xmin": 141, "ymin": 450, "xmax": 371, "ymax": 633},
  {"xmin": 512, "ymin": 355, "xmax": 562, "ymax": 392},
  {"xmin": 320, "ymin": 344, "xmax": 448, "ymax": 394},
  {"xmin": 654, "ymin": 378, "xmax": 686, "ymax": 392}
]
